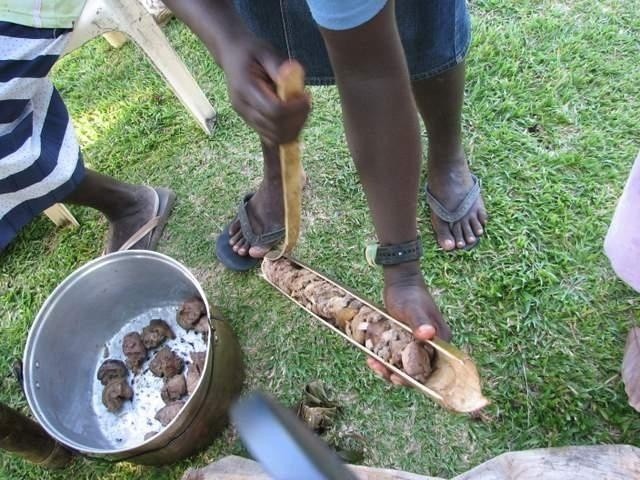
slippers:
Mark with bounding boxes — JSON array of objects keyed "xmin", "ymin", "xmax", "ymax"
[
  {"xmin": 426, "ymin": 173, "xmax": 480, "ymax": 252},
  {"xmin": 215, "ymin": 174, "xmax": 306, "ymax": 273},
  {"xmin": 100, "ymin": 187, "xmax": 176, "ymax": 256}
]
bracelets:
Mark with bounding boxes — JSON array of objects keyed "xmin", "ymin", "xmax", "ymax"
[{"xmin": 372, "ymin": 237, "xmax": 424, "ymax": 266}]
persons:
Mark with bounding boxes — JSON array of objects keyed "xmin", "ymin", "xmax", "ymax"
[
  {"xmin": 0, "ymin": 0, "xmax": 179, "ymax": 257},
  {"xmin": 161, "ymin": 1, "xmax": 490, "ymax": 391}
]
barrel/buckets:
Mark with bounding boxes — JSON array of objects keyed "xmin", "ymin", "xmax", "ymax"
[{"xmin": 14, "ymin": 250, "xmax": 244, "ymax": 465}]
